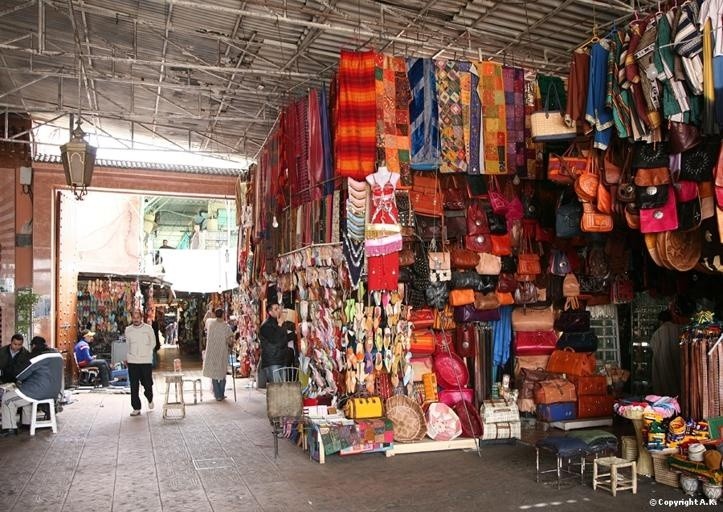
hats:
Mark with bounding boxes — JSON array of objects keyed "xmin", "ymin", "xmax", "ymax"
[
  {"xmin": 28, "ymin": 336, "xmax": 46, "ymax": 345},
  {"xmin": 81, "ymin": 328, "xmax": 96, "ymax": 337}
]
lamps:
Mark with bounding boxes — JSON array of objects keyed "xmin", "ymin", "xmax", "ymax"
[{"xmin": 59, "ymin": 119, "xmax": 97, "ymax": 202}]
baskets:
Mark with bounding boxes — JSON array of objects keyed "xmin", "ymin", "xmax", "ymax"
[
  {"xmin": 424, "ymin": 402, "xmax": 463, "ymax": 441},
  {"xmin": 383, "ymin": 393, "xmax": 428, "ymax": 444},
  {"xmin": 651, "ymin": 454, "xmax": 681, "ymax": 488},
  {"xmin": 455, "ymin": 399, "xmax": 484, "ymax": 438},
  {"xmin": 431, "ymin": 351, "xmax": 471, "ymax": 390},
  {"xmin": 621, "ymin": 435, "xmax": 638, "ymax": 461}
]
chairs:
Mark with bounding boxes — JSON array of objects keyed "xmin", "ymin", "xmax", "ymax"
[
  {"xmin": 265, "ymin": 383, "xmax": 313, "ymax": 460},
  {"xmin": 71, "ymin": 353, "xmax": 101, "ymax": 387}
]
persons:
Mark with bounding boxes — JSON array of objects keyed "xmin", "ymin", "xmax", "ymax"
[
  {"xmin": 124, "ymin": 309, "xmax": 156, "ymax": 416},
  {"xmin": 0, "ymin": 336, "xmax": 64, "ymax": 436},
  {"xmin": 202, "ymin": 308, "xmax": 234, "ymax": 400},
  {"xmin": 0, "ymin": 333, "xmax": 31, "ymax": 383},
  {"xmin": 160, "ymin": 240, "xmax": 176, "ymax": 248},
  {"xmin": 258, "ymin": 301, "xmax": 297, "ymax": 438},
  {"xmin": 76, "ymin": 328, "xmax": 119, "ymax": 388}
]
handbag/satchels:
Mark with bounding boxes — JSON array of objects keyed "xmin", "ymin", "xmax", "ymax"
[
  {"xmin": 537, "ymin": 404, "xmax": 576, "ymax": 421},
  {"xmin": 410, "ymin": 193, "xmax": 443, "ymax": 215},
  {"xmin": 535, "ymin": 223, "xmax": 552, "ymax": 241},
  {"xmin": 343, "ymin": 389, "xmax": 384, "ymax": 419},
  {"xmin": 427, "ymin": 283, "xmax": 448, "ymax": 310},
  {"xmin": 515, "ymin": 282, "xmax": 538, "ymax": 305},
  {"xmin": 604, "ymin": 145, "xmax": 625, "ymax": 184},
  {"xmin": 639, "ymin": 188, "xmax": 678, "ymax": 234},
  {"xmin": 427, "ymin": 251, "xmax": 450, "ymax": 270},
  {"xmin": 606, "ymin": 240, "xmax": 631, "ymax": 277},
  {"xmin": 409, "ymin": 290, "xmax": 426, "ymax": 309},
  {"xmin": 505, "ymin": 193, "xmax": 525, "ymax": 220},
  {"xmin": 456, "ymin": 323, "xmax": 476, "ymax": 358},
  {"xmin": 643, "ymin": 231, "xmax": 703, "ymax": 272},
  {"xmin": 574, "ymin": 145, "xmax": 598, "ymax": 201},
  {"xmin": 515, "ymin": 275, "xmax": 537, "ymax": 282},
  {"xmin": 444, "ymin": 176, "xmax": 465, "ymax": 210},
  {"xmin": 632, "ymin": 185, "xmax": 670, "ymax": 209},
  {"xmin": 400, "ymin": 242, "xmax": 415, "ymax": 266},
  {"xmin": 557, "ymin": 332, "xmax": 600, "ymax": 353},
  {"xmin": 410, "ymin": 310, "xmax": 435, "ymax": 330},
  {"xmin": 433, "ymin": 305, "xmax": 458, "ymax": 329},
  {"xmin": 475, "ymin": 253, "xmax": 502, "ymax": 275},
  {"xmin": 451, "ymin": 246, "xmax": 481, "ymax": 269},
  {"xmin": 680, "ymin": 148, "xmax": 716, "ymax": 182},
  {"xmin": 513, "ymin": 331, "xmax": 556, "ymax": 356},
  {"xmin": 618, "ymin": 148, "xmax": 636, "ymax": 203},
  {"xmin": 577, "ymin": 395, "xmax": 617, "ymax": 419},
  {"xmin": 577, "ymin": 274, "xmax": 597, "ymax": 294},
  {"xmin": 398, "ymin": 268, "xmax": 412, "ymax": 283},
  {"xmin": 588, "ymin": 279, "xmax": 611, "ymax": 305},
  {"xmin": 394, "ymin": 192, "xmax": 410, "ymax": 212},
  {"xmin": 518, "ymin": 232, "xmax": 541, "ymax": 275},
  {"xmin": 488, "ymin": 175, "xmax": 510, "ymax": 214},
  {"xmin": 562, "ymin": 274, "xmax": 581, "ymax": 312},
  {"xmin": 412, "ymin": 279, "xmax": 431, "ymax": 291},
  {"xmin": 479, "ymin": 274, "xmax": 499, "ymax": 293},
  {"xmin": 636, "ymin": 139, "xmax": 673, "ymax": 167},
  {"xmin": 515, "ymin": 367, "xmax": 567, "ymax": 399},
  {"xmin": 555, "ymin": 185, "xmax": 600, "ymax": 237},
  {"xmin": 513, "ymin": 357, "xmax": 549, "ymax": 380},
  {"xmin": 547, "ymin": 346, "xmax": 593, "ymax": 377},
  {"xmin": 498, "ymin": 274, "xmax": 518, "ymax": 293},
  {"xmin": 480, "ymin": 398, "xmax": 520, "ymax": 423},
  {"xmin": 468, "ymin": 202, "xmax": 491, "ymax": 236},
  {"xmin": 449, "ymin": 289, "xmax": 475, "ymax": 305},
  {"xmin": 410, "ymin": 330, "xmax": 435, "ymax": 354},
  {"xmin": 510, "ymin": 221, "xmax": 523, "ymax": 249},
  {"xmin": 612, "ymin": 278, "xmax": 634, "ymax": 304},
  {"xmin": 596, "ymin": 169, "xmax": 612, "ymax": 215},
  {"xmin": 413, "ymin": 242, "xmax": 429, "ymax": 278},
  {"xmin": 574, "ymin": 252, "xmax": 590, "ymax": 276},
  {"xmin": 501, "ymin": 255, "xmax": 517, "ymax": 273},
  {"xmin": 474, "ymin": 291, "xmax": 499, "ymax": 309},
  {"xmin": 429, "ymin": 270, "xmax": 451, "ymax": 282},
  {"xmin": 546, "ymin": 254, "xmax": 565, "ymax": 307},
  {"xmin": 523, "ymin": 219, "xmax": 536, "ymax": 239},
  {"xmin": 489, "ymin": 234, "xmax": 513, "ymax": 257},
  {"xmin": 454, "ymin": 304, "xmax": 500, "ymax": 322},
  {"xmin": 586, "ymin": 240, "xmax": 610, "ymax": 279},
  {"xmin": 512, "ymin": 304, "xmax": 555, "ymax": 332},
  {"xmin": 414, "ymin": 214, "xmax": 443, "ymax": 241},
  {"xmin": 546, "ymin": 249, "xmax": 572, "ymax": 276},
  {"xmin": 408, "ymin": 356, "xmax": 434, "ymax": 383},
  {"xmin": 413, "ymin": 170, "xmax": 439, "ymax": 195},
  {"xmin": 669, "ymin": 121, "xmax": 702, "ymax": 154},
  {"xmin": 634, "ymin": 169, "xmax": 670, "ymax": 187},
  {"xmin": 675, "ymin": 182, "xmax": 700, "ymax": 229},
  {"xmin": 465, "ymin": 234, "xmax": 493, "ymax": 252},
  {"xmin": 486, "ymin": 211, "xmax": 507, "ymax": 234},
  {"xmin": 495, "ymin": 289, "xmax": 515, "ymax": 305},
  {"xmin": 558, "ymin": 303, "xmax": 589, "ymax": 332},
  {"xmin": 534, "ymin": 375, "xmax": 577, "ymax": 404},
  {"xmin": 620, "ymin": 203, "xmax": 640, "ymax": 231},
  {"xmin": 445, "ymin": 209, "xmax": 468, "ymax": 241},
  {"xmin": 581, "ymin": 202, "xmax": 613, "ymax": 232},
  {"xmin": 478, "ymin": 422, "xmax": 522, "ymax": 440},
  {"xmin": 548, "ymin": 142, "xmax": 593, "ymax": 185},
  {"xmin": 446, "ymin": 266, "xmax": 480, "ymax": 290},
  {"xmin": 697, "ymin": 181, "xmax": 715, "ymax": 220},
  {"xmin": 537, "ymin": 240, "xmax": 551, "ymax": 256},
  {"xmin": 575, "ymin": 375, "xmax": 607, "ymax": 397}
]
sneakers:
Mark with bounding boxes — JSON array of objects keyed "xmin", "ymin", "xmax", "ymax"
[
  {"xmin": 148, "ymin": 401, "xmax": 154, "ymax": 409},
  {"xmin": 130, "ymin": 409, "xmax": 141, "ymax": 416}
]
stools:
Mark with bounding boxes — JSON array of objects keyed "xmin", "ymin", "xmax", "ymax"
[
  {"xmin": 176, "ymin": 377, "xmax": 200, "ymax": 405},
  {"xmin": 534, "ymin": 434, "xmax": 584, "ymax": 488},
  {"xmin": 164, "ymin": 372, "xmax": 185, "ymax": 416},
  {"xmin": 568, "ymin": 429, "xmax": 621, "ymax": 481},
  {"xmin": 28, "ymin": 399, "xmax": 56, "ymax": 435},
  {"xmin": 592, "ymin": 455, "xmax": 638, "ymax": 498}
]
